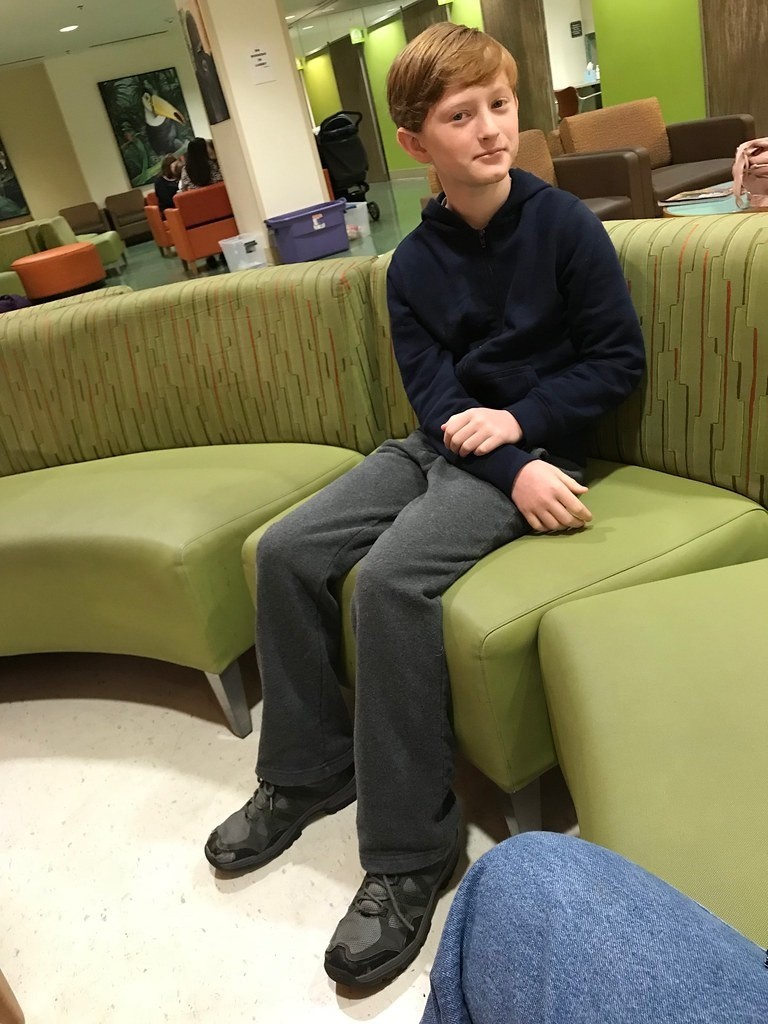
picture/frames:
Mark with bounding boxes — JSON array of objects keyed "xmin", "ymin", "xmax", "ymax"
[{"xmin": 96, "ymin": 67, "xmax": 195, "ymax": 188}]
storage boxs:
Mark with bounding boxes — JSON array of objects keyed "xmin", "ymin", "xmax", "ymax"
[
  {"xmin": 220, "ymin": 232, "xmax": 268, "ymax": 273},
  {"xmin": 344, "ymin": 201, "xmax": 370, "ymax": 241},
  {"xmin": 264, "ymin": 197, "xmax": 350, "ymax": 264}
]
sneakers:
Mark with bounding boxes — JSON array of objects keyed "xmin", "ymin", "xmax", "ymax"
[
  {"xmin": 205, "ymin": 764, "xmax": 357, "ymax": 872},
  {"xmin": 219, "ymin": 253, "xmax": 227, "ymax": 266},
  {"xmin": 324, "ymin": 830, "xmax": 462, "ymax": 988}
]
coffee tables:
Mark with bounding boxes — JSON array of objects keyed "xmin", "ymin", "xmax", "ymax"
[
  {"xmin": 11, "ymin": 242, "xmax": 107, "ymax": 305},
  {"xmin": 662, "ymin": 180, "xmax": 750, "ymax": 218}
]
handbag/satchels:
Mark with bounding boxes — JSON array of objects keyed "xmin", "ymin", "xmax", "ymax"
[
  {"xmin": 0, "ymin": 295, "xmax": 33, "ymax": 313},
  {"xmin": 732, "ymin": 137, "xmax": 768, "ymax": 211}
]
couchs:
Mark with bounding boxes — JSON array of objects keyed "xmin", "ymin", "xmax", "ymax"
[
  {"xmin": 144, "ymin": 192, "xmax": 175, "ymax": 260},
  {"xmin": 418, "ymin": 128, "xmax": 645, "ymax": 221},
  {"xmin": 239, "ymin": 210, "xmax": 768, "ymax": 830},
  {"xmin": 39, "ymin": 216, "xmax": 129, "ymax": 275},
  {"xmin": 105, "ymin": 189, "xmax": 150, "ymax": 241},
  {"xmin": 164, "ymin": 169, "xmax": 336, "ymax": 278},
  {"xmin": 59, "ymin": 202, "xmax": 111, "ymax": 236},
  {"xmin": 549, "ymin": 96, "xmax": 755, "ymax": 217},
  {"xmin": 534, "ymin": 559, "xmax": 768, "ymax": 946},
  {"xmin": 0, "ymin": 253, "xmax": 373, "ymax": 736}
]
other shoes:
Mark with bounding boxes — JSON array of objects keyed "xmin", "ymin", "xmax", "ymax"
[{"xmin": 207, "ymin": 256, "xmax": 218, "ymax": 269}]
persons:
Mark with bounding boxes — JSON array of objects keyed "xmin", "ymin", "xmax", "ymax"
[
  {"xmin": 179, "ymin": 136, "xmax": 229, "ymax": 271},
  {"xmin": 201, "ymin": 20, "xmax": 652, "ymax": 992},
  {"xmin": 418, "ymin": 829, "xmax": 767, "ymax": 1024},
  {"xmin": 154, "ymin": 153, "xmax": 182, "ymax": 220}
]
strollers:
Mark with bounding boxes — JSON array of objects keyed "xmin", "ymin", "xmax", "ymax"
[{"xmin": 311, "ymin": 110, "xmax": 381, "ymax": 222}]
